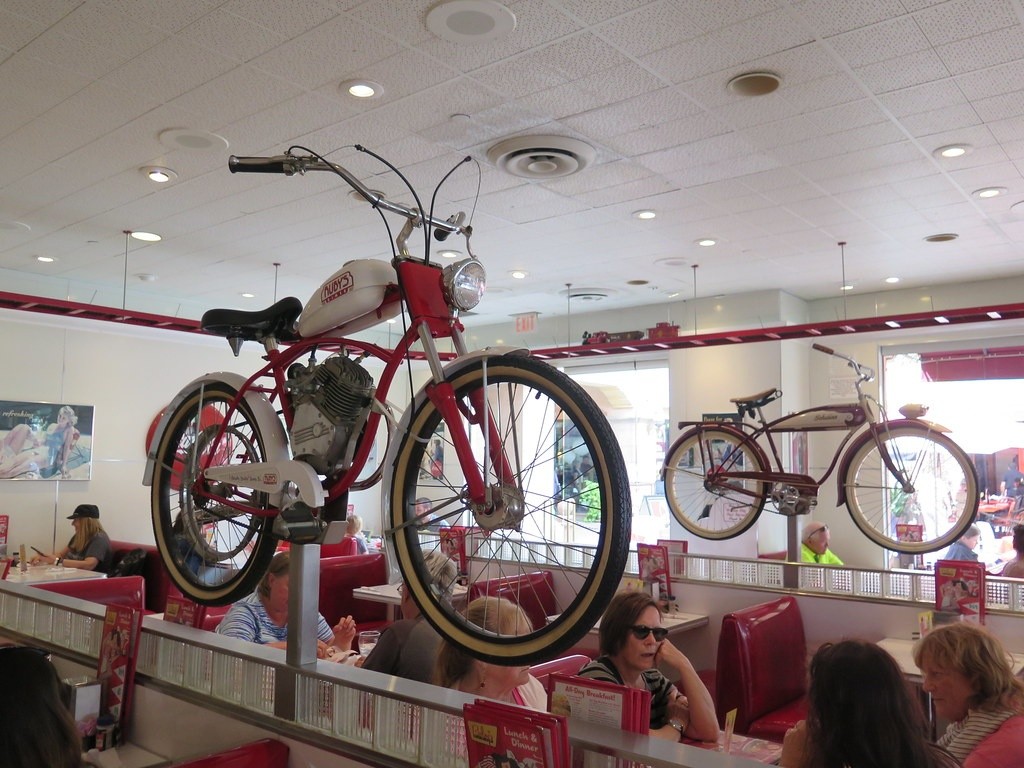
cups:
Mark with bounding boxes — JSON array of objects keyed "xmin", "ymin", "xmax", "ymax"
[{"xmin": 358, "ymin": 631, "xmax": 381, "ymax": 660}]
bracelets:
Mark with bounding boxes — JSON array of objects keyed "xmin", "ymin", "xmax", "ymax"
[{"xmin": 54, "ymin": 557, "xmax": 63, "ymax": 566}]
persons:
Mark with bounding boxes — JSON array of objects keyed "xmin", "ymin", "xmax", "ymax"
[
  {"xmin": 430, "ymin": 439, "xmax": 443, "ymax": 481},
  {"xmin": 28, "ymin": 503, "xmax": 116, "ymax": 576},
  {"xmin": 784, "ymin": 520, "xmax": 845, "ymax": 565},
  {"xmin": 213, "ymin": 550, "xmax": 357, "ymax": 662},
  {"xmin": 414, "ymin": 497, "xmax": 451, "ymax": 532},
  {"xmin": 576, "ymin": 588, "xmax": 721, "ymax": 745},
  {"xmin": 172, "ymin": 505, "xmax": 217, "ymax": 577},
  {"xmin": 0, "ymin": 405, "xmax": 77, "ymax": 479},
  {"xmin": 909, "ymin": 623, "xmax": 1024, "ymax": 768},
  {"xmin": 343, "ymin": 514, "xmax": 371, "ymax": 555},
  {"xmin": 1001, "ymin": 522, "xmax": 1024, "ymax": 580},
  {"xmin": 414, "ymin": 588, "xmax": 549, "ymax": 761},
  {"xmin": 776, "ymin": 637, "xmax": 962, "ymax": 768},
  {"xmin": 943, "ymin": 527, "xmax": 981, "ymax": 562},
  {"xmin": 354, "ymin": 549, "xmax": 463, "ymax": 688},
  {"xmin": 1001, "ymin": 460, "xmax": 1024, "ymax": 516},
  {"xmin": 0, "ymin": 646, "xmax": 99, "ymax": 768}
]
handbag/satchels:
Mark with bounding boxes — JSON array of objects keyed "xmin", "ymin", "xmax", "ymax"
[{"xmin": 108, "ymin": 549, "xmax": 156, "ymax": 604}]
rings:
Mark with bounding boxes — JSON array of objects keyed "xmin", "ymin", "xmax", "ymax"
[{"xmin": 37, "ymin": 560, "xmax": 40, "ymax": 562}]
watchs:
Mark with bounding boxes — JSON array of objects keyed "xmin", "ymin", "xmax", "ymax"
[{"xmin": 667, "ymin": 718, "xmax": 684, "ymax": 736}]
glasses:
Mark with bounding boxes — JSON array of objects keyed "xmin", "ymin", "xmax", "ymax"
[
  {"xmin": 808, "ymin": 526, "xmax": 827, "ymax": 538},
  {"xmin": 631, "ymin": 623, "xmax": 670, "ymax": 642},
  {"xmin": 396, "ymin": 583, "xmax": 407, "ymax": 597}
]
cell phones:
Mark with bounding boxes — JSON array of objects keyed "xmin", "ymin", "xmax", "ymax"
[{"xmin": 30, "ymin": 546, "xmax": 45, "ymax": 557}]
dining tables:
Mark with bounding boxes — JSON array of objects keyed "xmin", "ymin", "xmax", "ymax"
[
  {"xmin": 875, "ymin": 637, "xmax": 1024, "ymax": 739},
  {"xmin": 6, "ymin": 563, "xmax": 107, "ymax": 585},
  {"xmin": 547, "ymin": 610, "xmax": 709, "ymax": 637},
  {"xmin": 353, "ymin": 583, "xmax": 467, "ymax": 620}
]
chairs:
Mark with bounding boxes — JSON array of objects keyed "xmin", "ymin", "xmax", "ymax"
[
  {"xmin": 171, "ymin": 738, "xmax": 290, "ymax": 768},
  {"xmin": 35, "ymin": 536, "xmax": 231, "ymax": 633},
  {"xmin": 319, "ymin": 554, "xmax": 392, "ymax": 643},
  {"xmin": 468, "ymin": 571, "xmax": 556, "ymax": 630},
  {"xmin": 715, "ymin": 597, "xmax": 811, "ymax": 741},
  {"xmin": 533, "ymin": 655, "xmax": 590, "ymax": 692}
]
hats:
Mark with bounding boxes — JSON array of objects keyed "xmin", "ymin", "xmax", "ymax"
[{"xmin": 66, "ymin": 504, "xmax": 100, "ymax": 519}]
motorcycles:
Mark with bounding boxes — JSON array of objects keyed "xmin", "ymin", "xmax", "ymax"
[
  {"xmin": 143, "ymin": 143, "xmax": 632, "ymax": 666},
  {"xmin": 660, "ymin": 343, "xmax": 979, "ymax": 554}
]
